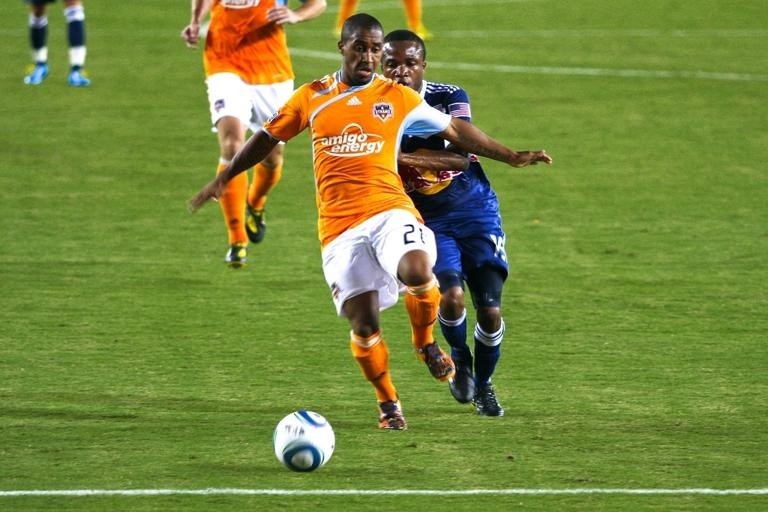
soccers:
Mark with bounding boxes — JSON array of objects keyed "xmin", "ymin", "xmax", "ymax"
[{"xmin": 271, "ymin": 410, "xmax": 336, "ymax": 473}]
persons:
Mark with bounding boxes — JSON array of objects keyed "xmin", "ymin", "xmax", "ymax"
[
  {"xmin": 23, "ymin": 0, "xmax": 90, "ymax": 87},
  {"xmin": 187, "ymin": 13, "xmax": 553, "ymax": 430},
  {"xmin": 380, "ymin": 29, "xmax": 508, "ymax": 418},
  {"xmin": 332, "ymin": 1, "xmax": 434, "ymax": 42},
  {"xmin": 180, "ymin": 0, "xmax": 328, "ymax": 268}
]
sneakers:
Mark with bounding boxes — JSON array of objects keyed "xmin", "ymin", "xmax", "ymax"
[
  {"xmin": 67, "ymin": 70, "xmax": 90, "ymax": 87},
  {"xmin": 23, "ymin": 64, "xmax": 50, "ymax": 85},
  {"xmin": 223, "ymin": 243, "xmax": 248, "ymax": 269},
  {"xmin": 412, "ymin": 338, "xmax": 456, "ymax": 382},
  {"xmin": 446, "ymin": 343, "xmax": 476, "ymax": 404},
  {"xmin": 376, "ymin": 392, "xmax": 408, "ymax": 431},
  {"xmin": 245, "ymin": 198, "xmax": 267, "ymax": 244},
  {"xmin": 470, "ymin": 376, "xmax": 505, "ymax": 417}
]
